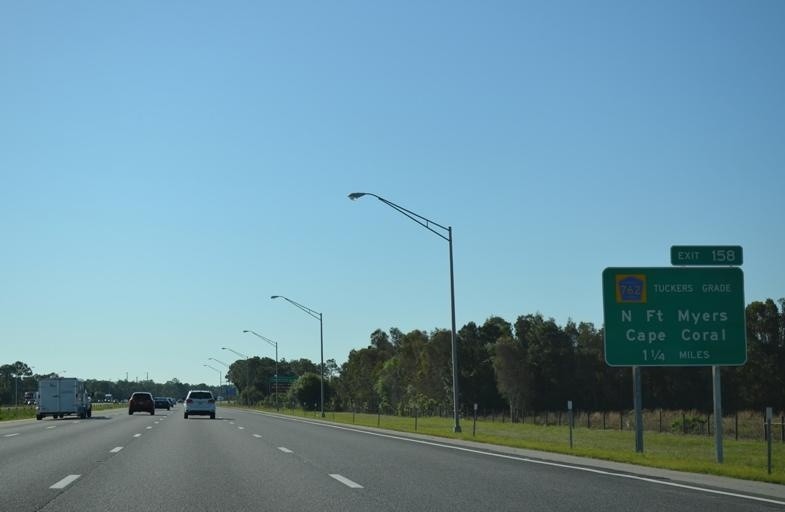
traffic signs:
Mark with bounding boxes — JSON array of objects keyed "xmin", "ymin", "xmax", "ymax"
[{"xmin": 602, "ymin": 266, "xmax": 747, "ymax": 366}]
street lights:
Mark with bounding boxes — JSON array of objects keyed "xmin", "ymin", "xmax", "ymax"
[
  {"xmin": 348, "ymin": 192, "xmax": 461, "ymax": 433},
  {"xmin": 15, "ymin": 361, "xmax": 32, "ymax": 407},
  {"xmin": 203, "ymin": 295, "xmax": 325, "ymax": 417}
]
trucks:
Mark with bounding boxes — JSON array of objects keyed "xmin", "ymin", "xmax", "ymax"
[
  {"xmin": 104, "ymin": 394, "xmax": 113, "ymax": 402},
  {"xmin": 36, "ymin": 378, "xmax": 93, "ymax": 420}
]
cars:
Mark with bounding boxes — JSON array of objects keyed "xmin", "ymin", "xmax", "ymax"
[{"xmin": 128, "ymin": 391, "xmax": 215, "ymax": 418}]
